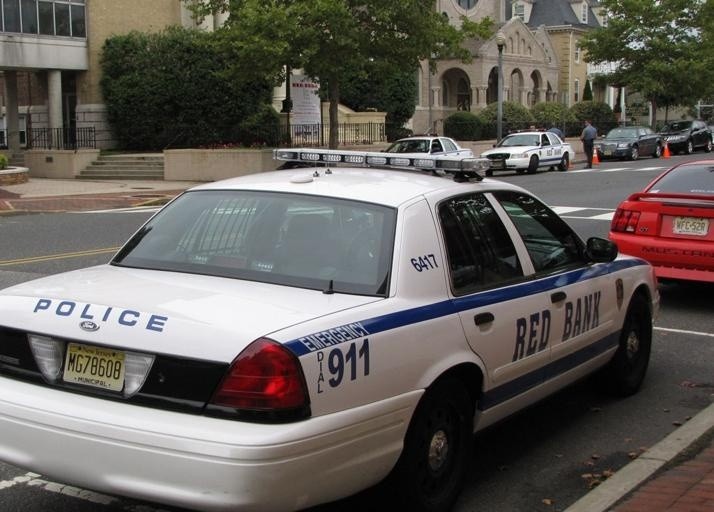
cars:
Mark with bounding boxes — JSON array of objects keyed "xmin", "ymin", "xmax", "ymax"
[
  {"xmin": 0, "ymin": 148, "xmax": 659, "ymax": 512},
  {"xmin": 593, "ymin": 126, "xmax": 661, "ymax": 160},
  {"xmin": 373, "ymin": 133, "xmax": 474, "ymax": 178},
  {"xmin": 707, "ymin": 125, "xmax": 714, "ymax": 145},
  {"xmin": 479, "ymin": 126, "xmax": 575, "ymax": 175},
  {"xmin": 608, "ymin": 159, "xmax": 714, "ymax": 294}
]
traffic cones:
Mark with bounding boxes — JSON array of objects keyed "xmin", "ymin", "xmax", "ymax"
[
  {"xmin": 590, "ymin": 145, "xmax": 601, "ymax": 164},
  {"xmin": 662, "ymin": 140, "xmax": 673, "ymax": 159}
]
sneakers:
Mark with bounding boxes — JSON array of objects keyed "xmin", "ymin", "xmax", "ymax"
[{"xmin": 584, "ymin": 165, "xmax": 592, "ymax": 169}]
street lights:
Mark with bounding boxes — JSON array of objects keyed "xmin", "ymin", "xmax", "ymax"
[{"xmin": 494, "ymin": 29, "xmax": 508, "ymax": 147}]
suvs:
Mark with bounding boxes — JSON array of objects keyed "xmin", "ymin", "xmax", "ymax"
[{"xmin": 656, "ymin": 119, "xmax": 713, "ymax": 153}]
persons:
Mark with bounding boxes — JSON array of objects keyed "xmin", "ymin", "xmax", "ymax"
[{"xmin": 546, "ymin": 119, "xmax": 598, "ymax": 171}]
